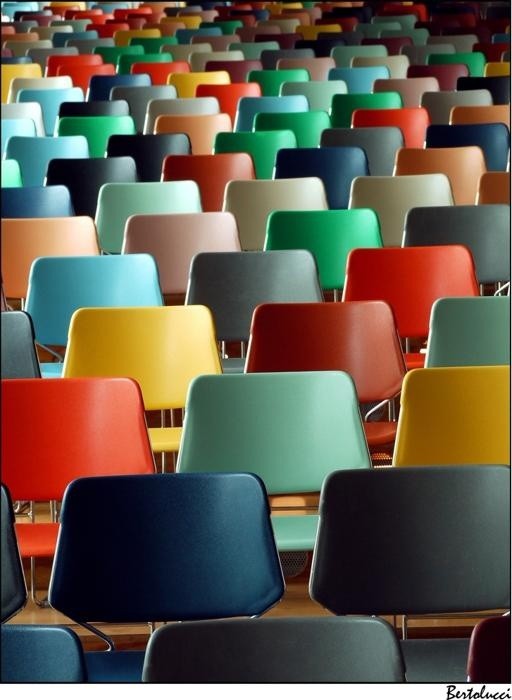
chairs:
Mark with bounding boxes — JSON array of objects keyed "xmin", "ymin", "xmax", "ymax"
[{"xmin": 0, "ymin": 0, "xmax": 511, "ymax": 683}]
